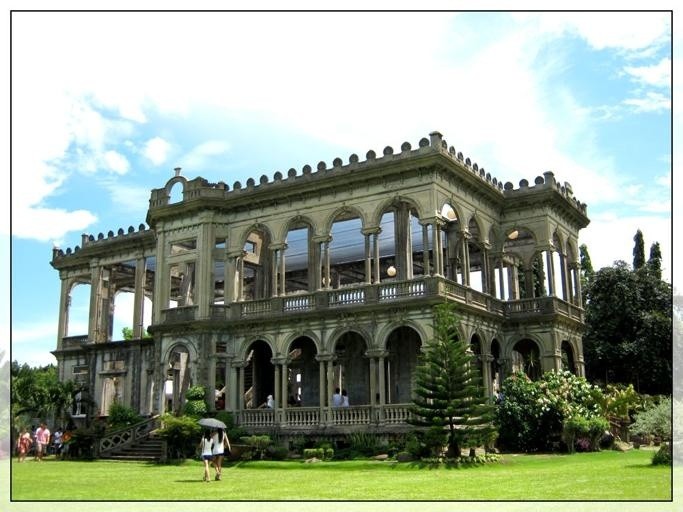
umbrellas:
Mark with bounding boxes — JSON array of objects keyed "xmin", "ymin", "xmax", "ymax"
[{"xmin": 196, "ymin": 417, "xmax": 227, "ymax": 428}]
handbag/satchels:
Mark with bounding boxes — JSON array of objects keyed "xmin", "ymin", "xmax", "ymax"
[{"xmin": 224, "ymin": 448, "xmax": 230, "ymax": 457}]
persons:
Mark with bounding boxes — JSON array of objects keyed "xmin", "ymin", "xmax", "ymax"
[
  {"xmin": 258, "ymin": 395, "xmax": 275, "ymax": 408},
  {"xmin": 199, "ymin": 429, "xmax": 213, "ymax": 482},
  {"xmin": 333, "ymin": 387, "xmax": 349, "ymax": 406},
  {"xmin": 16, "ymin": 422, "xmax": 63, "ymax": 462},
  {"xmin": 213, "ymin": 428, "xmax": 231, "ymax": 480}
]
